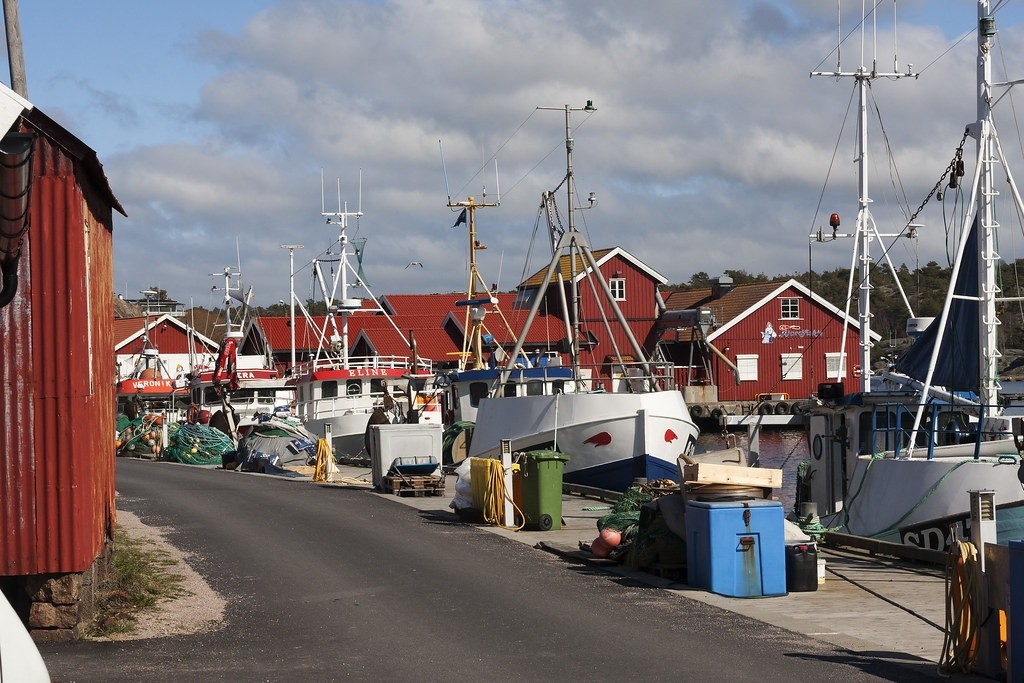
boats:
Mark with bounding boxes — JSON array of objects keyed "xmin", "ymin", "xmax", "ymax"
[
  {"xmin": 274, "ymin": 166, "xmax": 446, "ymax": 465},
  {"xmin": 719, "ymin": 0, "xmax": 1023, "ymax": 550},
  {"xmin": 115, "ymin": 235, "xmax": 299, "ymax": 434},
  {"xmin": 441, "ymin": 101, "xmax": 699, "ymax": 500}
]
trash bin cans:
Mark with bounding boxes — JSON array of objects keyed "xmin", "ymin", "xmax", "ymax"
[{"xmin": 513, "ymin": 450, "xmax": 571, "ymax": 531}]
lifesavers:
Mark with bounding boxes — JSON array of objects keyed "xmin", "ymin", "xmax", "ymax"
[
  {"xmin": 759, "ymin": 403, "xmax": 773, "ymax": 414},
  {"xmin": 711, "ymin": 408, "xmax": 722, "ymax": 420},
  {"xmin": 186, "ymin": 406, "xmax": 199, "ymax": 424},
  {"xmin": 691, "ymin": 405, "xmax": 702, "ymax": 416},
  {"xmin": 776, "ymin": 402, "xmax": 789, "ymax": 415},
  {"xmin": 791, "ymin": 402, "xmax": 805, "ymax": 414}
]
